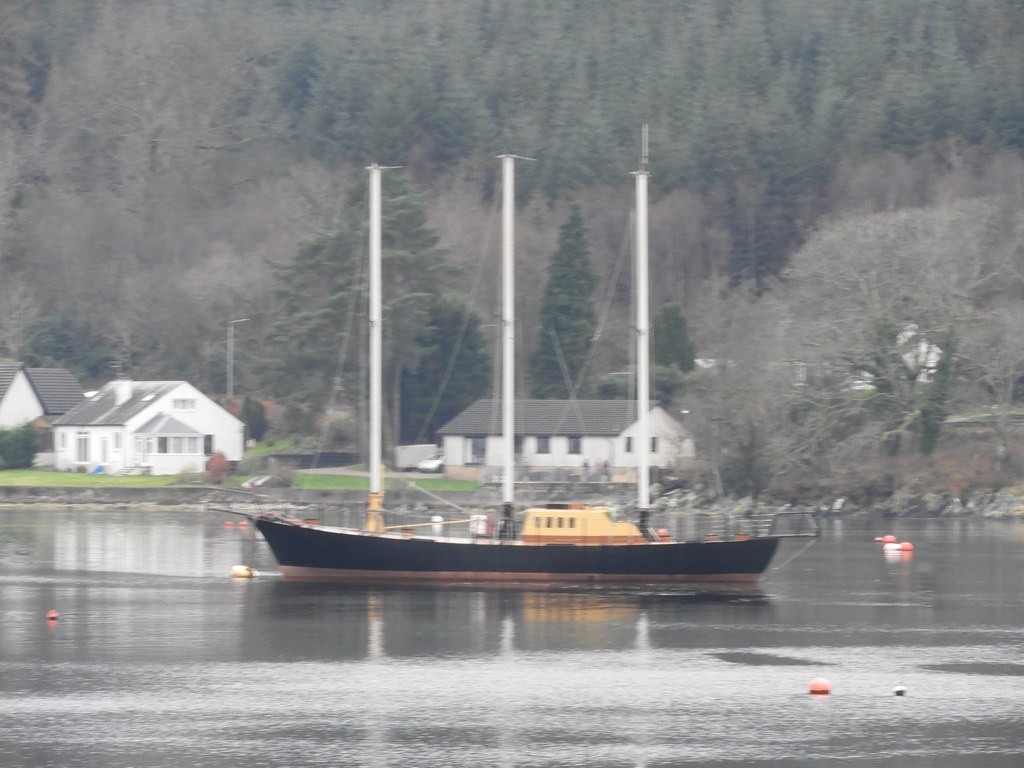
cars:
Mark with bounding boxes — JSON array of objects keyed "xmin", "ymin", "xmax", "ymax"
[
  {"xmin": 240, "ymin": 475, "xmax": 291, "ymax": 489},
  {"xmin": 416, "ymin": 452, "xmax": 443, "ymax": 474}
]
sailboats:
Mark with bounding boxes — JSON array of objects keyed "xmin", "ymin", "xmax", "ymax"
[{"xmin": 204, "ymin": 124, "xmax": 820, "ymax": 596}]
